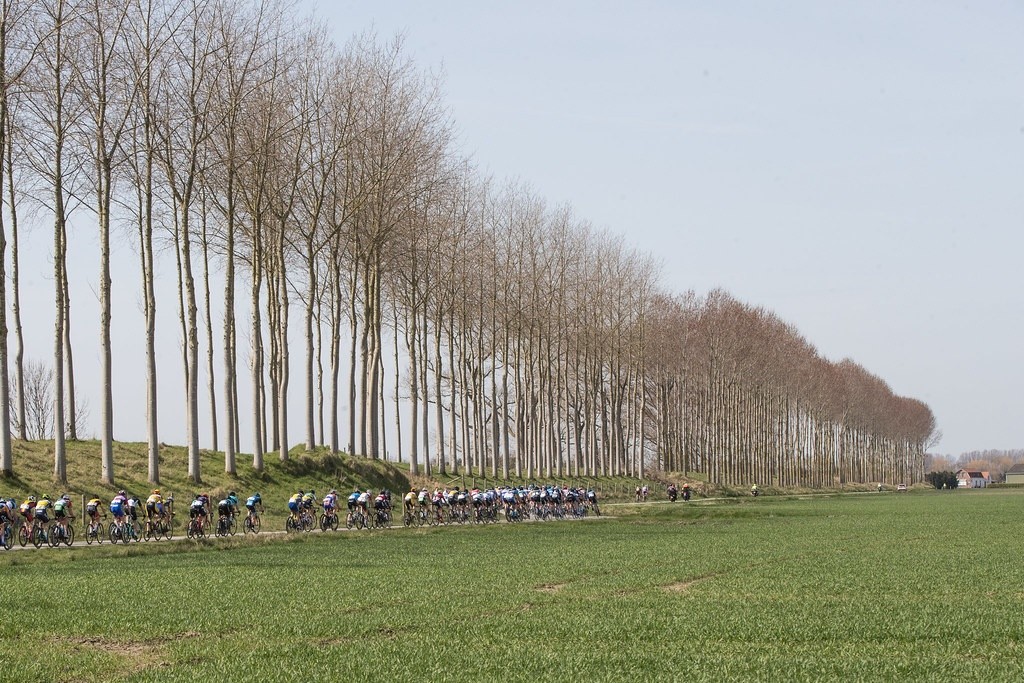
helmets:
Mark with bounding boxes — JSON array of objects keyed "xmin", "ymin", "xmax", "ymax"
[
  {"xmin": 229, "ymin": 491, "xmax": 235, "ymax": 495},
  {"xmin": 202, "ymin": 493, "xmax": 208, "ymax": 498},
  {"xmin": 298, "ymin": 486, "xmax": 459, "ymax": 494},
  {"xmin": 0, "ymin": 489, "xmax": 174, "ymax": 502},
  {"xmin": 255, "ymin": 493, "xmax": 259, "ymax": 496}
]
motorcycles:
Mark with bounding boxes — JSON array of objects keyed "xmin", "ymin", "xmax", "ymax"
[
  {"xmin": 669, "ymin": 490, "xmax": 678, "ymax": 502},
  {"xmin": 751, "ymin": 489, "xmax": 759, "ymax": 497},
  {"xmin": 681, "ymin": 489, "xmax": 692, "ymax": 501},
  {"xmin": 878, "ymin": 487, "xmax": 881, "ymax": 492}
]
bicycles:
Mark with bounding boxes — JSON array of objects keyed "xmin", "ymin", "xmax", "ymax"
[
  {"xmin": 590, "ymin": 500, "xmax": 601, "ymax": 517},
  {"xmin": 319, "ymin": 510, "xmax": 340, "ymax": 531},
  {"xmin": 215, "ymin": 511, "xmax": 240, "ymax": 538},
  {"xmin": 85, "ymin": 515, "xmax": 106, "ymax": 545},
  {"xmin": 243, "ymin": 510, "xmax": 264, "ymax": 534},
  {"xmin": 187, "ymin": 513, "xmax": 212, "ymax": 539},
  {"xmin": 643, "ymin": 492, "xmax": 648, "ymax": 503},
  {"xmin": 286, "ymin": 508, "xmax": 318, "ymax": 532},
  {"xmin": 345, "ymin": 505, "xmax": 395, "ymax": 530},
  {"xmin": 0, "ymin": 517, "xmax": 77, "ymax": 550},
  {"xmin": 636, "ymin": 493, "xmax": 641, "ymax": 503},
  {"xmin": 108, "ymin": 514, "xmax": 142, "ymax": 543},
  {"xmin": 404, "ymin": 503, "xmax": 501, "ymax": 527},
  {"xmin": 143, "ymin": 513, "xmax": 175, "ymax": 541},
  {"xmin": 505, "ymin": 500, "xmax": 588, "ymax": 522}
]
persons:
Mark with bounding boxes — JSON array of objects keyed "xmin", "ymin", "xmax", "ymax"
[
  {"xmin": 110, "ymin": 489, "xmax": 176, "ymax": 539},
  {"xmin": 667, "ymin": 484, "xmax": 678, "ymax": 500},
  {"xmin": 190, "ymin": 494, "xmax": 214, "ymax": 535},
  {"xmin": 752, "ymin": 484, "xmax": 759, "ymax": 495},
  {"xmin": 0, "ymin": 494, "xmax": 76, "ymax": 546},
  {"xmin": 86, "ymin": 494, "xmax": 107, "ymax": 538},
  {"xmin": 878, "ymin": 483, "xmax": 881, "ymax": 490},
  {"xmin": 682, "ymin": 484, "xmax": 690, "ymax": 499},
  {"xmin": 348, "ymin": 490, "xmax": 390, "ymax": 527},
  {"xmin": 405, "ymin": 484, "xmax": 599, "ymax": 519},
  {"xmin": 218, "ymin": 492, "xmax": 241, "ymax": 533},
  {"xmin": 636, "ymin": 485, "xmax": 648, "ymax": 498},
  {"xmin": 289, "ymin": 490, "xmax": 341, "ymax": 528},
  {"xmin": 246, "ymin": 494, "xmax": 264, "ymax": 533}
]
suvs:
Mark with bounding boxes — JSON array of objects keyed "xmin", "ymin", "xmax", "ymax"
[{"xmin": 898, "ymin": 484, "xmax": 907, "ymax": 491}]
